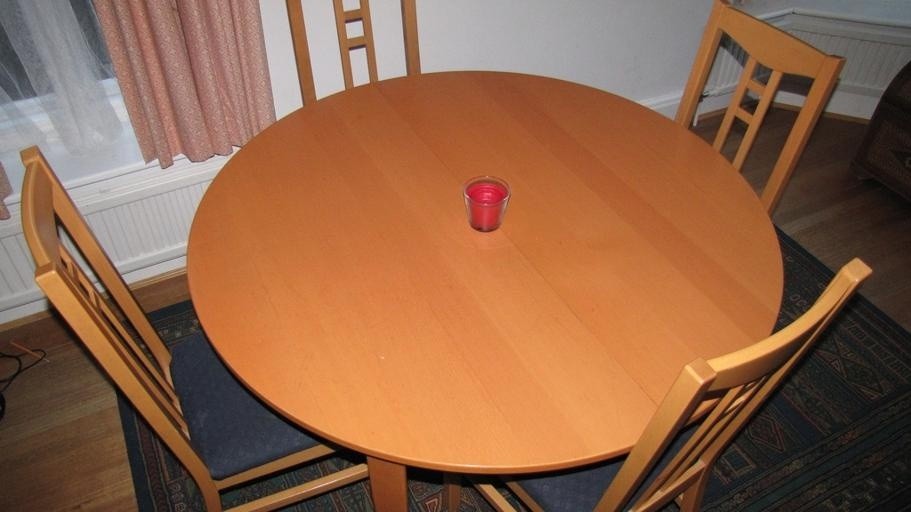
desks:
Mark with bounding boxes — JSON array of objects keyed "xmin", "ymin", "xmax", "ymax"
[{"xmin": 184, "ymin": 70, "xmax": 785, "ymax": 512}]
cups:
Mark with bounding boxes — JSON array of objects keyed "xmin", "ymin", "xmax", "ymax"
[{"xmin": 464, "ymin": 176, "xmax": 511, "ymax": 231}]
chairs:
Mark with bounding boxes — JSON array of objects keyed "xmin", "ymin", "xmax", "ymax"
[
  {"xmin": 21, "ymin": 143, "xmax": 370, "ymax": 510},
  {"xmin": 671, "ymin": 0, "xmax": 847, "ymax": 219},
  {"xmin": 285, "ymin": 0, "xmax": 421, "ymax": 108},
  {"xmin": 470, "ymin": 257, "xmax": 875, "ymax": 512}
]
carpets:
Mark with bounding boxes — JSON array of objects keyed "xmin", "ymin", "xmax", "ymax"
[{"xmin": 106, "ymin": 221, "xmax": 911, "ymax": 512}]
